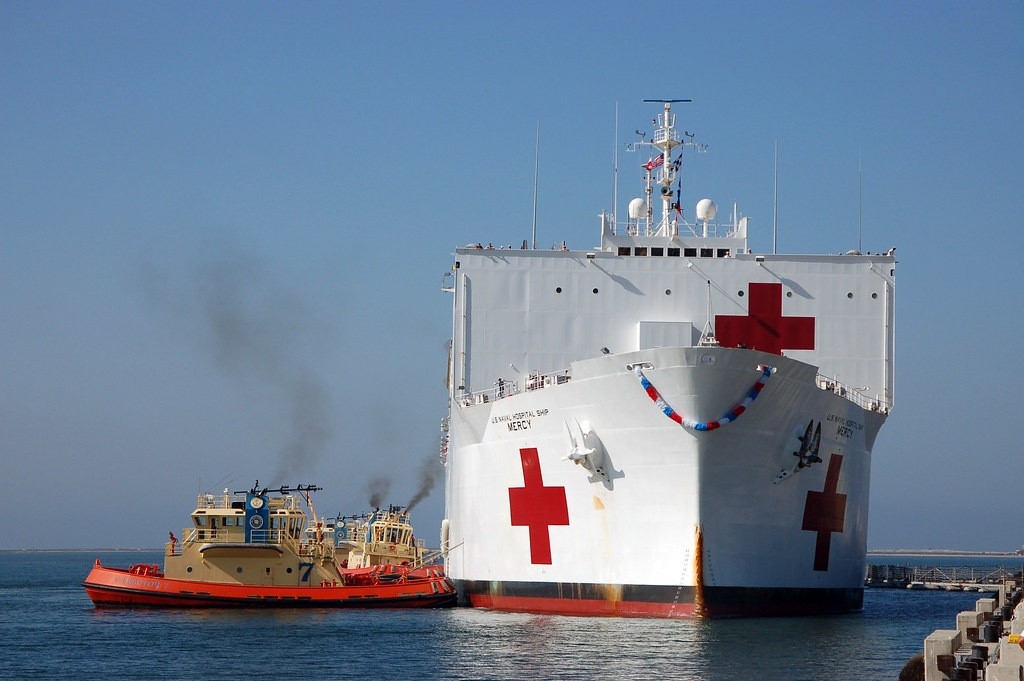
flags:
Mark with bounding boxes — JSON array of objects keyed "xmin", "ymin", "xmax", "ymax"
[{"xmin": 646, "ymin": 152, "xmax": 682, "ymax": 220}]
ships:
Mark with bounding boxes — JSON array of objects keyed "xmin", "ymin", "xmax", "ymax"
[{"xmin": 442, "ymin": 97, "xmax": 898, "ymax": 622}]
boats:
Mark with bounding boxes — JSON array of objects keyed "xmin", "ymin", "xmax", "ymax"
[{"xmin": 82, "ymin": 480, "xmax": 460, "ymax": 608}]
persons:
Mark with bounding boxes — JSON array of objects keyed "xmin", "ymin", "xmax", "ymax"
[
  {"xmin": 387, "ymin": 530, "xmax": 415, "ymax": 547},
  {"xmin": 737, "ymin": 343, "xmax": 747, "ymax": 349},
  {"xmin": 498, "ymin": 378, "xmax": 504, "ymax": 397}
]
